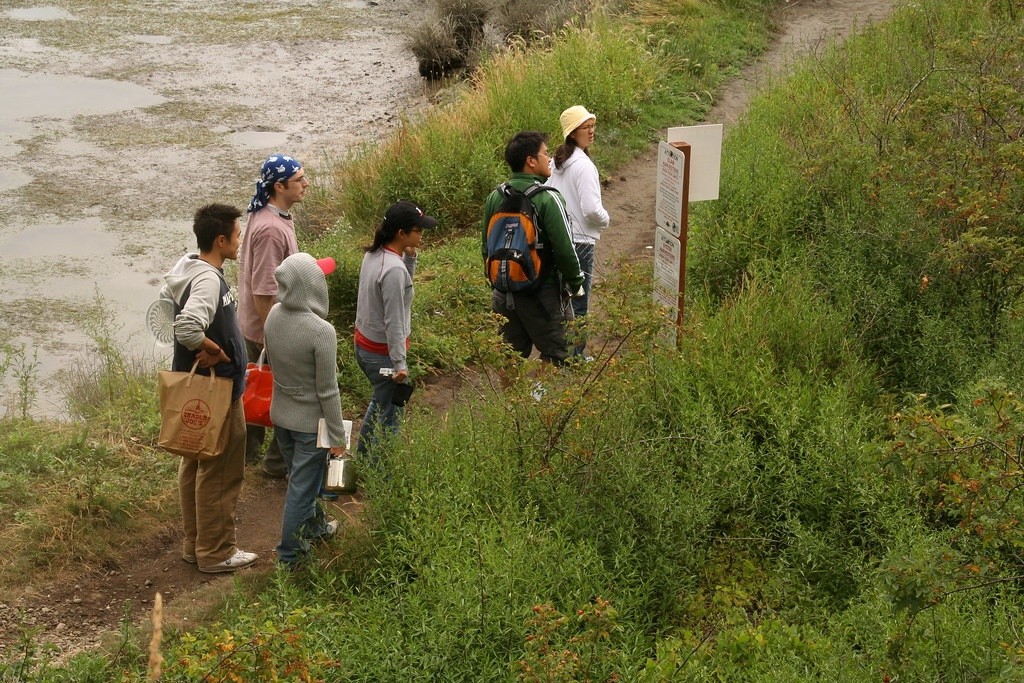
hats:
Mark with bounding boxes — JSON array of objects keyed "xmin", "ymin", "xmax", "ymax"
[
  {"xmin": 560, "ymin": 105, "xmax": 596, "ymax": 141},
  {"xmin": 316, "ymin": 257, "xmax": 336, "ymax": 274},
  {"xmin": 383, "ymin": 202, "xmax": 439, "ymax": 228}
]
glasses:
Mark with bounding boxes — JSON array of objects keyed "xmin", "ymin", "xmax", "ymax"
[
  {"xmin": 579, "ymin": 122, "xmax": 595, "ymax": 130},
  {"xmin": 409, "ymin": 228, "xmax": 423, "ymax": 235},
  {"xmin": 533, "ymin": 150, "xmax": 551, "ymax": 160},
  {"xmin": 280, "ymin": 175, "xmax": 307, "ymax": 183}
]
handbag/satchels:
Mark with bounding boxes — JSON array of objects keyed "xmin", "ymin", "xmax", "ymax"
[
  {"xmin": 243, "ymin": 348, "xmax": 273, "ymax": 426},
  {"xmin": 157, "ymin": 360, "xmax": 234, "ymax": 461}
]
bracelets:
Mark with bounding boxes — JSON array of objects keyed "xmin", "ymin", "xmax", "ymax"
[{"xmin": 206, "ymin": 349, "xmax": 221, "ymax": 356}]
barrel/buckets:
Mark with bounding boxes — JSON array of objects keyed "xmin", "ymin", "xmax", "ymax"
[{"xmin": 322, "ymin": 449, "xmax": 358, "ymax": 495}]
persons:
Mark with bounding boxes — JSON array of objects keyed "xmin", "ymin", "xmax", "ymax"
[
  {"xmin": 355, "ymin": 200, "xmax": 440, "ymax": 474},
  {"xmin": 160, "ymin": 204, "xmax": 259, "ymax": 574},
  {"xmin": 235, "ymin": 152, "xmax": 307, "ymax": 468},
  {"xmin": 262, "ymin": 253, "xmax": 346, "ymax": 571},
  {"xmin": 544, "ymin": 105, "xmax": 610, "ymax": 363},
  {"xmin": 481, "ymin": 130, "xmax": 585, "ymax": 370}
]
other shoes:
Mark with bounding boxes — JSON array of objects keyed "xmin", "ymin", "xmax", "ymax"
[
  {"xmin": 259, "ymin": 466, "xmax": 289, "ymax": 479},
  {"xmin": 583, "ymin": 356, "xmax": 594, "ymax": 363},
  {"xmin": 528, "ymin": 381, "xmax": 548, "ymax": 402},
  {"xmin": 319, "ymin": 490, "xmax": 339, "ymax": 501},
  {"xmin": 318, "ymin": 519, "xmax": 340, "ymax": 544}
]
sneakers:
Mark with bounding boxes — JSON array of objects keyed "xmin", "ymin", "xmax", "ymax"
[
  {"xmin": 183, "ymin": 553, "xmax": 197, "ymax": 562},
  {"xmin": 198, "ymin": 548, "xmax": 258, "ymax": 572}
]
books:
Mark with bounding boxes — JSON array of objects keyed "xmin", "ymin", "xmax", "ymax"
[{"xmin": 316, "ymin": 418, "xmax": 352, "ymax": 449}]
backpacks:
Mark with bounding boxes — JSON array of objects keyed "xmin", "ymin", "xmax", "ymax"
[{"xmin": 485, "ymin": 182, "xmax": 569, "ymax": 310}]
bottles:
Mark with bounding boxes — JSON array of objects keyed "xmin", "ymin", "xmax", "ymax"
[{"xmin": 316, "ymin": 257, "xmax": 336, "ymax": 276}]
